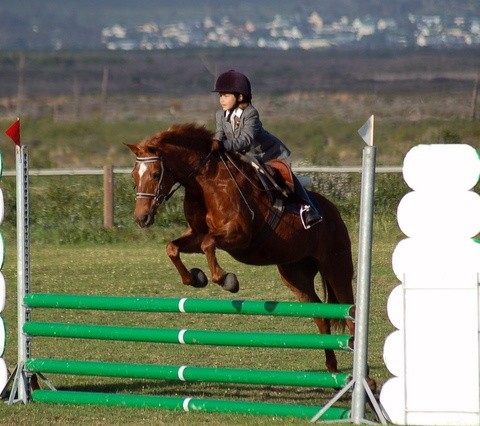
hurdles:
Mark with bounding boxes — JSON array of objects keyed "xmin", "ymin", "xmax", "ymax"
[{"xmin": 0, "ymin": 115, "xmax": 391, "ymax": 426}]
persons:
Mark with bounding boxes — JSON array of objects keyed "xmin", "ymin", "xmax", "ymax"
[{"xmin": 208, "ymin": 69, "xmax": 322, "ymax": 226}]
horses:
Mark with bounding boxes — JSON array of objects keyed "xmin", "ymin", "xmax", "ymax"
[{"xmin": 123, "ymin": 121, "xmax": 378, "ymax": 397}]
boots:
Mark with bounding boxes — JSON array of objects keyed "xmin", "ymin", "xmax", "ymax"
[{"xmin": 290, "ymin": 171, "xmax": 319, "ymax": 225}]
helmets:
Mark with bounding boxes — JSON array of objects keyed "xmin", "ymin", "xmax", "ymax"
[{"xmin": 212, "ymin": 69, "xmax": 251, "ymax": 96}]
